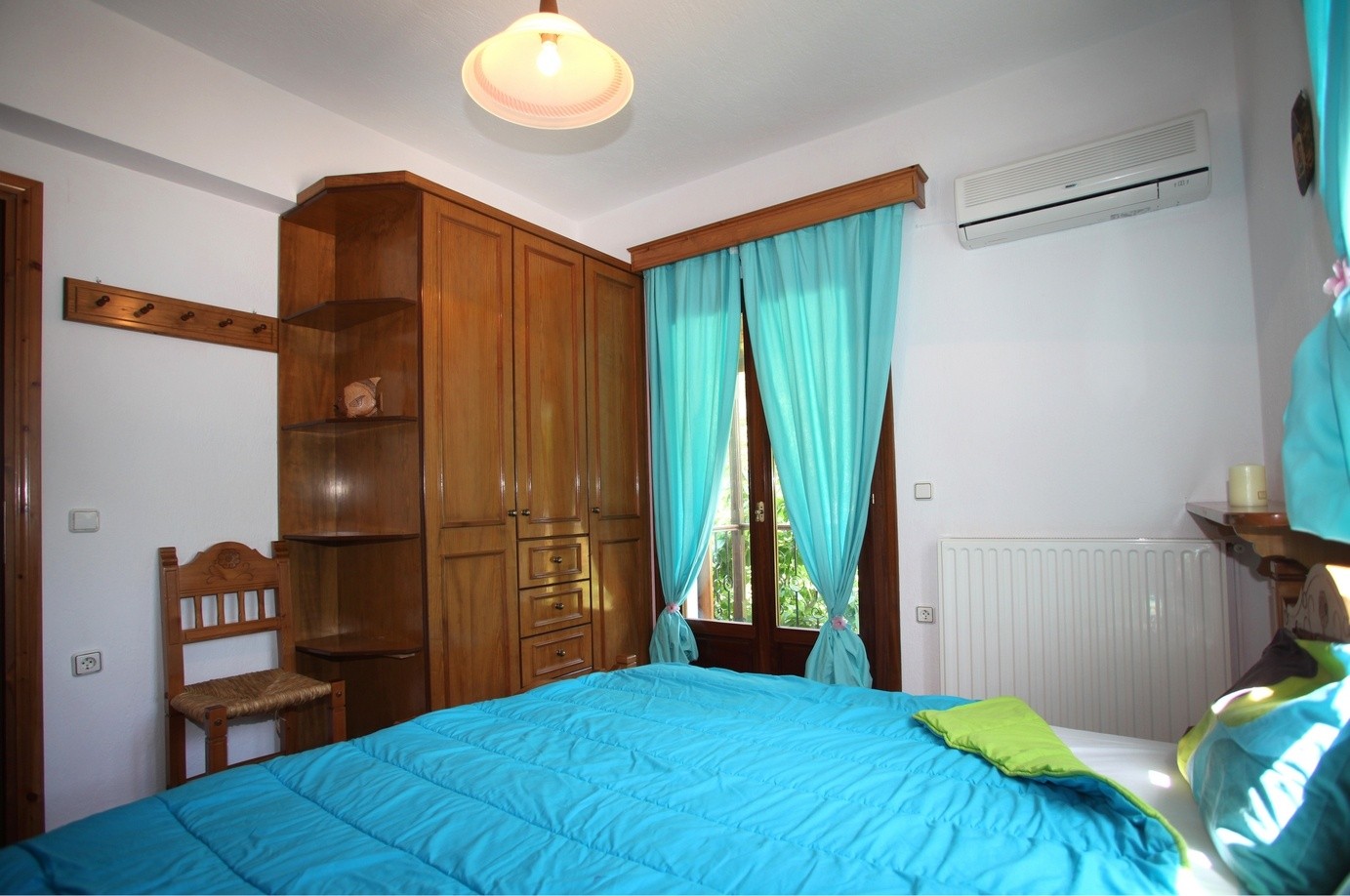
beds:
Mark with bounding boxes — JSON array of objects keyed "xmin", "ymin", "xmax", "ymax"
[{"xmin": 0, "ymin": 550, "xmax": 1350, "ymax": 896}]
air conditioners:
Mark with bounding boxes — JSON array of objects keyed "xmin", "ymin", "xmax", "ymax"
[{"xmin": 947, "ymin": 106, "xmax": 1222, "ymax": 254}]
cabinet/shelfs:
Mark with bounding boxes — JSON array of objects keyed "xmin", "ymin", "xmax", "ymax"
[{"xmin": 274, "ymin": 169, "xmax": 655, "ymax": 750}]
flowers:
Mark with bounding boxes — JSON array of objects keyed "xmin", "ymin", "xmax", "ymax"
[
  {"xmin": 661, "ymin": 601, "xmax": 681, "ymax": 615},
  {"xmin": 1320, "ymin": 255, "xmax": 1350, "ymax": 299},
  {"xmin": 828, "ymin": 614, "xmax": 851, "ymax": 631}
]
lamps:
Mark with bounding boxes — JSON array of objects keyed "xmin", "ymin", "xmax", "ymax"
[{"xmin": 457, "ymin": 0, "xmax": 641, "ymax": 139}]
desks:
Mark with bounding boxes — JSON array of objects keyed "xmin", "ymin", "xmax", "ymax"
[{"xmin": 1184, "ymin": 494, "xmax": 1350, "ymax": 576}]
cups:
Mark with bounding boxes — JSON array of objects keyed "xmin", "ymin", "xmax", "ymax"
[{"xmin": 1229, "ymin": 465, "xmax": 1267, "ymax": 507}]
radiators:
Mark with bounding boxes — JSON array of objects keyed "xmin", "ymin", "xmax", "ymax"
[{"xmin": 934, "ymin": 532, "xmax": 1235, "ymax": 744}]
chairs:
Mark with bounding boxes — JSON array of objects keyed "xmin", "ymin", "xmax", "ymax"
[{"xmin": 150, "ymin": 539, "xmax": 350, "ymax": 792}]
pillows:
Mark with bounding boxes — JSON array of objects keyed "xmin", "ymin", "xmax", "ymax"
[{"xmin": 1171, "ymin": 625, "xmax": 1350, "ymax": 896}]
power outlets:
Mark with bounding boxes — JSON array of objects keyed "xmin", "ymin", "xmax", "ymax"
[
  {"xmin": 914, "ymin": 604, "xmax": 935, "ymax": 625},
  {"xmin": 69, "ymin": 649, "xmax": 105, "ymax": 678}
]
